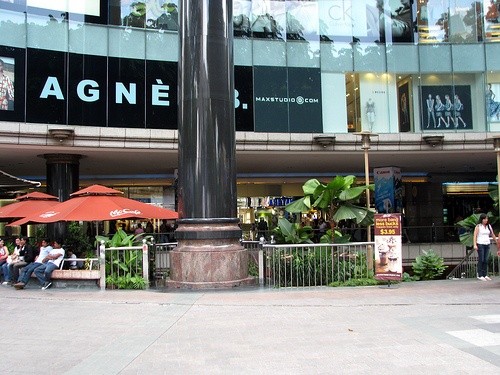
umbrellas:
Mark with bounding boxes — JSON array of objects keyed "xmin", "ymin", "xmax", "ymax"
[{"xmin": 0, "ymin": 184, "xmax": 179, "ymax": 258}]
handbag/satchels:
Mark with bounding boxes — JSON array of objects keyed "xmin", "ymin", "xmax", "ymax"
[{"xmin": 6, "ymin": 255, "xmax": 25, "ymax": 264}]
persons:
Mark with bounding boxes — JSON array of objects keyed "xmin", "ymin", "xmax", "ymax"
[
  {"xmin": 473, "ymin": 213, "xmax": 498, "ymax": 281},
  {"xmin": 0, "ymin": 237, "xmax": 84, "ymax": 291},
  {"xmin": 146, "ymin": 218, "xmax": 154, "ymax": 233},
  {"xmin": 251, "ymin": 217, "xmax": 267, "ymax": 241},
  {"xmin": 487, "ymin": 83, "xmax": 500, "ymax": 121},
  {"xmin": 317, "ymin": 218, "xmax": 327, "ymax": 243},
  {"xmin": 149, "ymin": 3, "xmax": 180, "ymax": 30},
  {"xmin": 122, "ymin": 2, "xmax": 145, "ymax": 28},
  {"xmin": 134, "ymin": 223, "xmax": 144, "ymax": 241},
  {"xmin": 359, "ymin": 0, "xmax": 415, "ymax": 41},
  {"xmin": 118, "ymin": 219, "xmax": 126, "ymax": 231},
  {"xmin": 426, "ymin": 93, "xmax": 466, "ymax": 128},
  {"xmin": 366, "ymin": 97, "xmax": 376, "ymax": 133},
  {"xmin": 160, "ymin": 219, "xmax": 171, "ymax": 243},
  {"xmin": 0, "ymin": 58, "xmax": 14, "ymax": 110}
]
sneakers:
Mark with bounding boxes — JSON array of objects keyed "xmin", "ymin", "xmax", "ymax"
[{"xmin": 42, "ymin": 282, "xmax": 51, "ymax": 290}]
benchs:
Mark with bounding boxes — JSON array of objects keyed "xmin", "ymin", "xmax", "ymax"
[{"xmin": 19, "ymin": 258, "xmax": 100, "ymax": 280}]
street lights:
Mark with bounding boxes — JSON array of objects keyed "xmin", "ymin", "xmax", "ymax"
[{"xmin": 352, "ymin": 130, "xmax": 379, "ymax": 242}]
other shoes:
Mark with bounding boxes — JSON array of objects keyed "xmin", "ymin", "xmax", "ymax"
[
  {"xmin": 479, "ymin": 276, "xmax": 491, "ymax": 281},
  {"xmin": 0, "ymin": 281, "xmax": 25, "ymax": 289}
]
